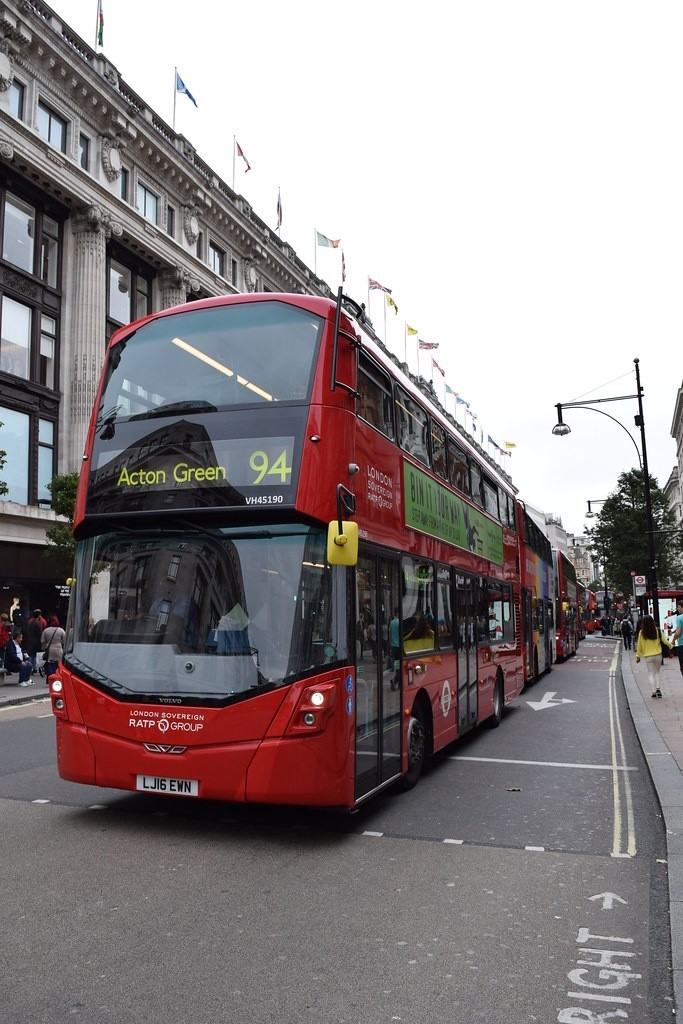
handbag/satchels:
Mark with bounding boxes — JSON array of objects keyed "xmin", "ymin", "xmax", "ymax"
[
  {"xmin": 35, "ymin": 652, "xmax": 47, "ymax": 670},
  {"xmin": 662, "ymin": 643, "xmax": 670, "ymax": 658},
  {"xmin": 43, "ymin": 653, "xmax": 48, "ymax": 660}
]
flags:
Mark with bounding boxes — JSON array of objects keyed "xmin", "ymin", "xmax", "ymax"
[
  {"xmin": 369, "ymin": 278, "xmax": 517, "ymax": 458},
  {"xmin": 274, "ymin": 195, "xmax": 282, "ymax": 231},
  {"xmin": 177, "ymin": 73, "xmax": 197, "ymax": 109},
  {"xmin": 317, "ymin": 232, "xmax": 341, "ymax": 247},
  {"xmin": 237, "ymin": 144, "xmax": 251, "ymax": 173},
  {"xmin": 99, "ymin": 3, "xmax": 103, "ymax": 48}
]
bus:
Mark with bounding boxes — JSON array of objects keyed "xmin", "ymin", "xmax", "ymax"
[
  {"xmin": 552, "ymin": 549, "xmax": 580, "ymax": 663},
  {"xmin": 576, "ymin": 581, "xmax": 615, "ymax": 641},
  {"xmin": 47, "ymin": 287, "xmax": 524, "ymax": 814},
  {"xmin": 516, "ymin": 498, "xmax": 557, "ymax": 687}
]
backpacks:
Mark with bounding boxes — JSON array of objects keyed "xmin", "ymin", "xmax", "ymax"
[{"xmin": 622, "ymin": 621, "xmax": 631, "ymax": 636}]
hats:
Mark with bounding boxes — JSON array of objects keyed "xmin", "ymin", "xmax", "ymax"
[
  {"xmin": 34, "ymin": 609, "xmax": 41, "ymax": 612},
  {"xmin": 49, "ymin": 616, "xmax": 60, "ymax": 627}
]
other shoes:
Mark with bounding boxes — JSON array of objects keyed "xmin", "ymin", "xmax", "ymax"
[
  {"xmin": 390, "ymin": 679, "xmax": 399, "ymax": 691},
  {"xmin": 18, "ymin": 681, "xmax": 28, "ymax": 687},
  {"xmin": 39, "ymin": 667, "xmax": 45, "ymax": 677},
  {"xmin": 652, "ymin": 695, "xmax": 656, "ymax": 697},
  {"xmin": 656, "ymin": 690, "xmax": 662, "ymax": 698},
  {"xmin": 26, "ymin": 679, "xmax": 36, "ymax": 685}
]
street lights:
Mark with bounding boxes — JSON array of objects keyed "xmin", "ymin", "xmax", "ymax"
[{"xmin": 552, "ymin": 358, "xmax": 683, "ymax": 665}]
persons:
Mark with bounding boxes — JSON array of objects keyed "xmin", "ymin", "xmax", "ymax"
[
  {"xmin": 0, "ymin": 613, "xmax": 12, "ymax": 676},
  {"xmin": 620, "ymin": 616, "xmax": 633, "ymax": 650},
  {"xmin": 424, "ymin": 606, "xmax": 434, "ymax": 625},
  {"xmin": 388, "ymin": 607, "xmax": 403, "ymax": 691},
  {"xmin": 358, "ymin": 606, "xmax": 388, "ymax": 664},
  {"xmin": 404, "ymin": 615, "xmax": 442, "ymax": 664},
  {"xmin": 26, "ymin": 609, "xmax": 47, "ymax": 676},
  {"xmin": 636, "ymin": 615, "xmax": 675, "ymax": 699},
  {"xmin": 10, "ymin": 597, "xmax": 24, "ymax": 636},
  {"xmin": 6, "ymin": 633, "xmax": 36, "ymax": 687},
  {"xmin": 41, "ymin": 616, "xmax": 65, "ymax": 686},
  {"xmin": 672, "ymin": 600, "xmax": 683, "ymax": 679},
  {"xmin": 601, "ymin": 614, "xmax": 615, "ymax": 638},
  {"xmin": 448, "ymin": 615, "xmax": 498, "ymax": 649}
]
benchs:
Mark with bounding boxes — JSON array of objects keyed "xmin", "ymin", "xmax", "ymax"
[{"xmin": 0, "ymin": 668, "xmax": 33, "ymax": 686}]
